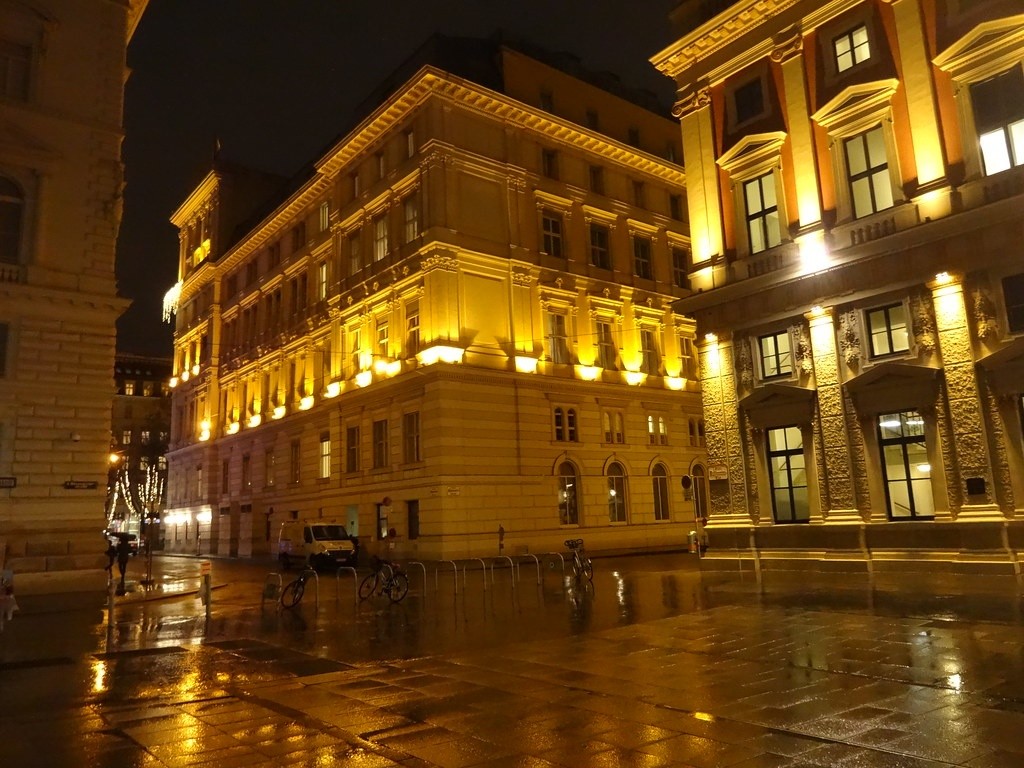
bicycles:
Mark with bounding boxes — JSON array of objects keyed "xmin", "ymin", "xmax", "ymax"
[
  {"xmin": 357, "ymin": 554, "xmax": 411, "ymax": 603},
  {"xmin": 564, "ymin": 537, "xmax": 594, "ymax": 581},
  {"xmin": 279, "ymin": 564, "xmax": 316, "ymax": 609}
]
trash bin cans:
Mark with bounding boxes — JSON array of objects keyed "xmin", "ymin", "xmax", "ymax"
[{"xmin": 687, "ymin": 529, "xmax": 697, "ymax": 554}]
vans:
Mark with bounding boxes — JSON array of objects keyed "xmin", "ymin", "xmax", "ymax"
[{"xmin": 277, "ymin": 517, "xmax": 356, "ymax": 569}]
